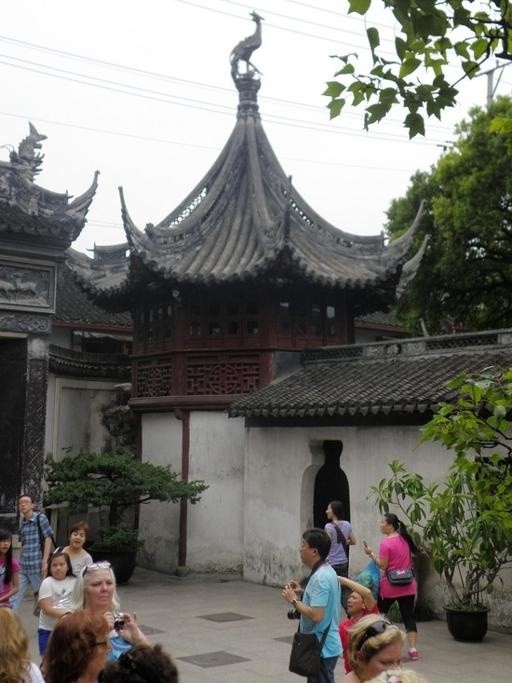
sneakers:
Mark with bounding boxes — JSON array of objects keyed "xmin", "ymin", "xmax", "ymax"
[{"xmin": 406, "ymin": 652, "xmax": 418, "ymax": 659}]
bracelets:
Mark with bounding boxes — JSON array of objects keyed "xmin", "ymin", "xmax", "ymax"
[{"xmin": 369, "ymin": 552, "xmax": 373, "ymax": 558}]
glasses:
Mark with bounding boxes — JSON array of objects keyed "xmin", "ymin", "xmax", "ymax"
[
  {"xmin": 83, "ymin": 562, "xmax": 112, "ymax": 578},
  {"xmin": 354, "ymin": 617, "xmax": 392, "ymax": 650}
]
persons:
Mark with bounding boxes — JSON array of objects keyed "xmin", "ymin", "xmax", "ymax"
[
  {"xmin": 0, "ymin": 522, "xmax": 179, "ymax": 683},
  {"xmin": 281, "ymin": 528, "xmax": 344, "ymax": 683},
  {"xmin": 362, "ymin": 513, "xmax": 419, "ymax": 660},
  {"xmin": 337, "ymin": 575, "xmax": 427, "ymax": 683},
  {"xmin": 7, "ymin": 495, "xmax": 55, "ymax": 616},
  {"xmin": 324, "ymin": 501, "xmax": 356, "ymax": 615}
]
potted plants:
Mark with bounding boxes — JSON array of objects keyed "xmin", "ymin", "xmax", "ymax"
[
  {"xmin": 364, "ymin": 463, "xmax": 511, "ymax": 642},
  {"xmin": 42, "ymin": 448, "xmax": 210, "ymax": 587}
]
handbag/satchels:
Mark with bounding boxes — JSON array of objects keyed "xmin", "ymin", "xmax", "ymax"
[
  {"xmin": 289, "ymin": 631, "xmax": 324, "ymax": 677},
  {"xmin": 387, "ymin": 569, "xmax": 413, "ymax": 586}
]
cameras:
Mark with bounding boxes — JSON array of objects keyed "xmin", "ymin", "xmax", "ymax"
[
  {"xmin": 287, "ymin": 609, "xmax": 300, "ymax": 619},
  {"xmin": 112, "ymin": 611, "xmax": 124, "ymax": 630}
]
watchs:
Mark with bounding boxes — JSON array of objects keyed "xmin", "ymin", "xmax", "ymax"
[{"xmin": 292, "ymin": 598, "xmax": 299, "ymax": 606}]
